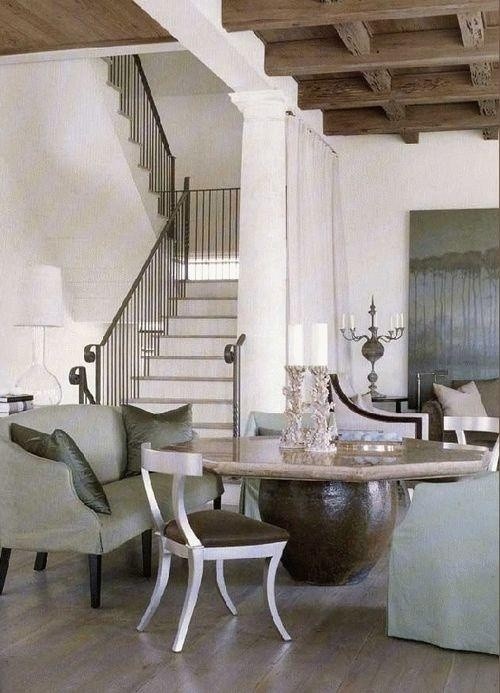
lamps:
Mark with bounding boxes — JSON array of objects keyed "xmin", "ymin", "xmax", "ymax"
[{"xmin": 9, "ymin": 261, "xmax": 66, "ymax": 405}]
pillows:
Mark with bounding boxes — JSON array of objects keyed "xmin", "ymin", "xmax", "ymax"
[
  {"xmin": 8, "ymin": 422, "xmax": 112, "ymax": 515},
  {"xmin": 432, "ymin": 381, "xmax": 487, "ymax": 417},
  {"xmin": 363, "ymin": 392, "xmax": 372, "ymax": 410},
  {"xmin": 349, "ymin": 394, "xmax": 362, "ymax": 408},
  {"xmin": 119, "ymin": 402, "xmax": 193, "ymax": 477}
]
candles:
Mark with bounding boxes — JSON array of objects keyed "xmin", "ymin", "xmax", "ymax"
[
  {"xmin": 390, "ymin": 316, "xmax": 393, "ymax": 329},
  {"xmin": 400, "ymin": 311, "xmax": 405, "ymax": 327},
  {"xmin": 395, "ymin": 312, "xmax": 399, "ymax": 326},
  {"xmin": 342, "ymin": 312, "xmax": 344, "ymax": 329},
  {"xmin": 351, "ymin": 313, "xmax": 355, "ymax": 328},
  {"xmin": 371, "ymin": 294, "xmax": 374, "ymax": 306}
]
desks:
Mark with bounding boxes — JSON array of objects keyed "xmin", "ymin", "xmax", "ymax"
[{"xmin": 157, "ymin": 435, "xmax": 489, "ymax": 585}]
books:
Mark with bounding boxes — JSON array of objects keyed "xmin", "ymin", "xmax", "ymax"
[
  {"xmin": 0, "ymin": 394, "xmax": 34, "ymax": 412},
  {"xmin": 332, "ymin": 430, "xmax": 402, "ymax": 442}
]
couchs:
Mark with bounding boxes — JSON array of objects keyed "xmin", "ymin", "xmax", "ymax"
[
  {"xmin": 0, "ymin": 405, "xmax": 224, "ymax": 608},
  {"xmin": 237, "ymin": 413, "xmax": 337, "ymax": 518},
  {"xmin": 422, "ymin": 379, "xmax": 500, "ymax": 442},
  {"xmin": 387, "ymin": 471, "xmax": 500, "ymax": 627}
]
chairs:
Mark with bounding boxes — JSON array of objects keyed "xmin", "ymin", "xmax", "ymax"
[{"xmin": 137, "ymin": 443, "xmax": 293, "ymax": 646}]
